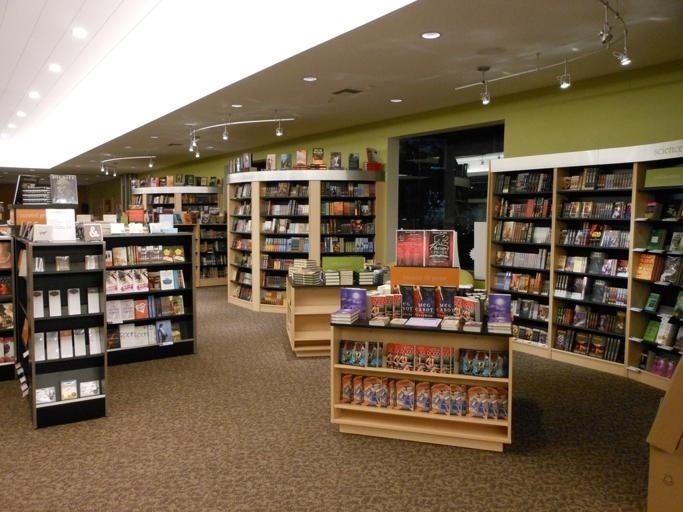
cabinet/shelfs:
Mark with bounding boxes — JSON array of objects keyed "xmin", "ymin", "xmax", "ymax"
[
  {"xmin": 329, "ymin": 319, "xmax": 512, "ymax": 452},
  {"xmin": 0, "ymin": 232, "xmax": 197, "ymax": 382},
  {"xmin": 286, "ymin": 274, "xmax": 383, "ymax": 358},
  {"xmin": 12, "ymin": 235, "xmax": 109, "ymax": 430},
  {"xmin": 225, "ymin": 170, "xmax": 385, "ymax": 314},
  {"xmin": 131, "ymin": 186, "xmax": 227, "ymax": 287},
  {"xmin": 486, "ymin": 138, "xmax": 683, "ymax": 392}
]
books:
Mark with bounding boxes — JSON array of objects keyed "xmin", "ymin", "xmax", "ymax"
[{"xmin": 0, "ymin": 147, "xmax": 683, "ymax": 422}]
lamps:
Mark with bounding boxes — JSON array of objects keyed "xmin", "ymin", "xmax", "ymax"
[
  {"xmin": 100, "ymin": 156, "xmax": 157, "ymax": 177},
  {"xmin": 455, "ymin": 0, "xmax": 631, "ymax": 105},
  {"xmin": 189, "ymin": 109, "xmax": 295, "ymax": 159}
]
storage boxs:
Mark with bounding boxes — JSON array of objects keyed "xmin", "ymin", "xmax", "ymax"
[{"xmin": 8, "ymin": 204, "xmax": 78, "ymax": 225}]
